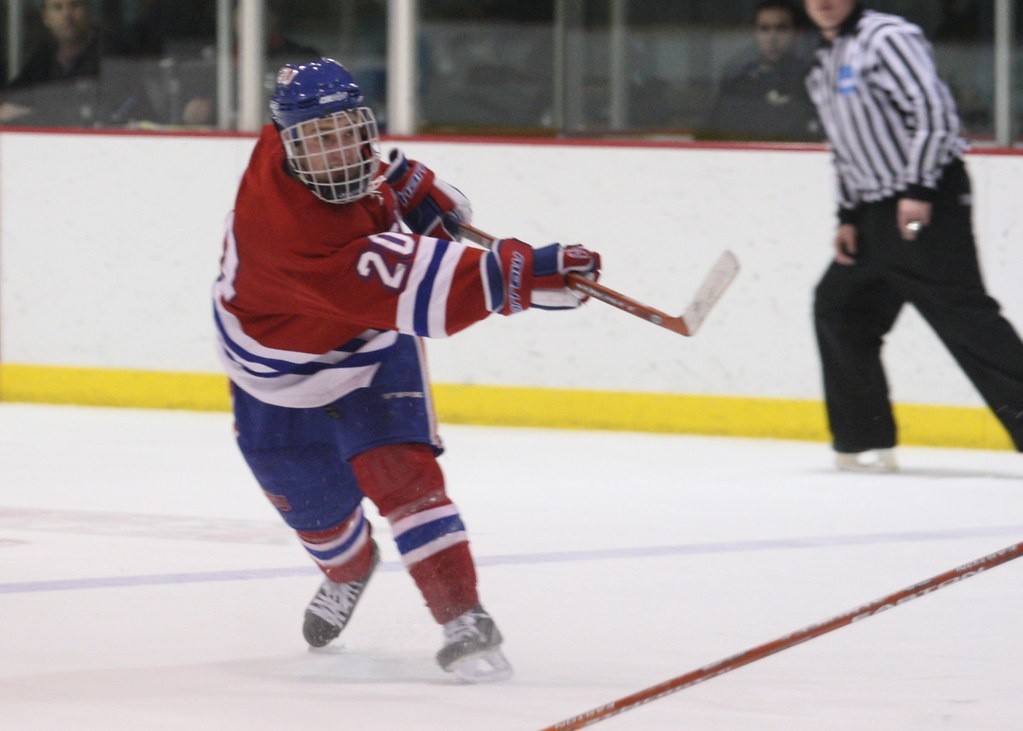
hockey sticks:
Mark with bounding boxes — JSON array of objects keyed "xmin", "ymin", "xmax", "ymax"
[
  {"xmin": 538, "ymin": 540, "xmax": 1023, "ymax": 731},
  {"xmin": 448, "ymin": 216, "xmax": 741, "ymax": 339}
]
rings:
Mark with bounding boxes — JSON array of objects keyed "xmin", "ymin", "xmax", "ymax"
[{"xmin": 907, "ymin": 218, "xmax": 922, "ymax": 232}]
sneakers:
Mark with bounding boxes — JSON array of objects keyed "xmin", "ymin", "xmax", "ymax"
[
  {"xmin": 302, "ymin": 536, "xmax": 379, "ymax": 648},
  {"xmin": 435, "ymin": 603, "xmax": 513, "ymax": 683}
]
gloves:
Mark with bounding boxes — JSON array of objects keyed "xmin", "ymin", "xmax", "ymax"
[
  {"xmin": 402, "ymin": 175, "xmax": 472, "ymax": 243},
  {"xmin": 529, "ymin": 243, "xmax": 601, "ymax": 311}
]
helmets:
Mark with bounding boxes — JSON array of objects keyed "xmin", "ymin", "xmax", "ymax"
[{"xmin": 270, "ymin": 56, "xmax": 369, "ymax": 200}]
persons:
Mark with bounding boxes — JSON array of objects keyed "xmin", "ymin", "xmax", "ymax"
[
  {"xmin": 700, "ymin": 0, "xmax": 827, "ymax": 141},
  {"xmin": 802, "ymin": 0, "xmax": 1023, "ymax": 470},
  {"xmin": 214, "ymin": 56, "xmax": 602, "ymax": 683},
  {"xmin": 0, "ymin": 0, "xmax": 320, "ymax": 132}
]
